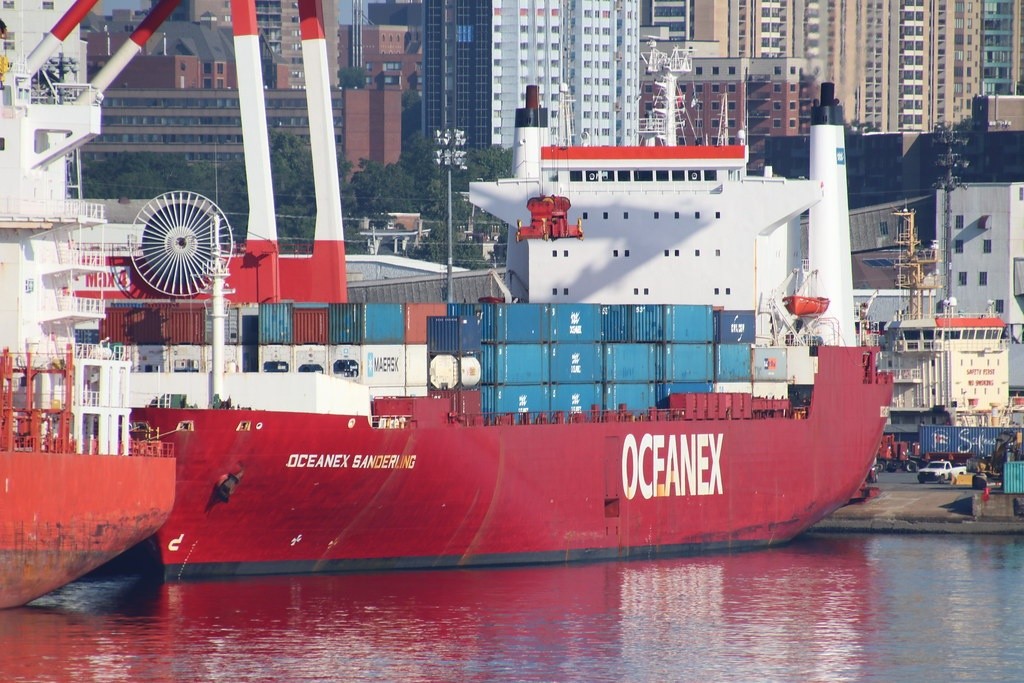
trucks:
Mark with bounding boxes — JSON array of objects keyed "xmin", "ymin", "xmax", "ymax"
[{"xmin": 885, "ymin": 424, "xmax": 1024, "ymax": 473}]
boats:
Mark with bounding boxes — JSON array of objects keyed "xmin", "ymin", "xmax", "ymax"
[
  {"xmin": 783, "ymin": 295, "xmax": 830, "ymax": 320},
  {"xmin": 0, "ymin": 0, "xmax": 896, "ymax": 611}
]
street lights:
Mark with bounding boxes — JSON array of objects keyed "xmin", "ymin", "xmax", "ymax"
[{"xmin": 436, "ymin": 125, "xmax": 467, "ymax": 301}]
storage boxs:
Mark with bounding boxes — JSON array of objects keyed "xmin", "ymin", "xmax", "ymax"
[
  {"xmin": 1004, "ymin": 461, "xmax": 1024, "ymax": 493},
  {"xmin": 76, "ymin": 299, "xmax": 789, "ymax": 424},
  {"xmin": 916, "ymin": 422, "xmax": 1024, "ymax": 455}
]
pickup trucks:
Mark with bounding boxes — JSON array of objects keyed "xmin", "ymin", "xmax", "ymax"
[{"xmin": 917, "ymin": 459, "xmax": 967, "ymax": 484}]
